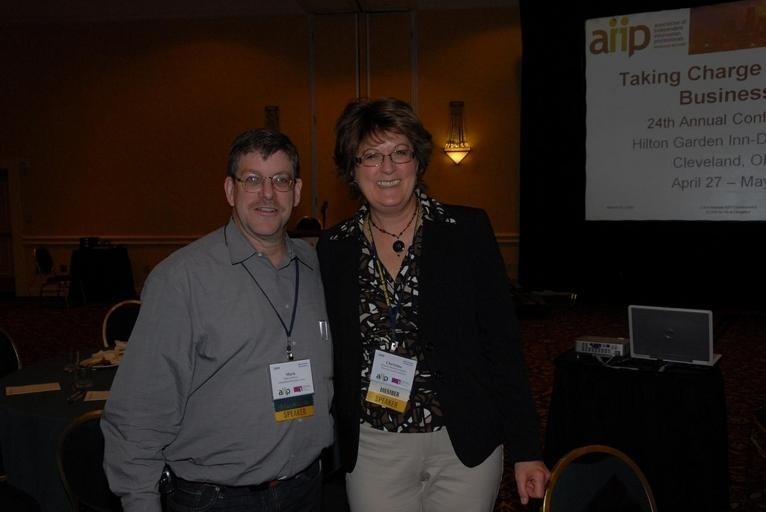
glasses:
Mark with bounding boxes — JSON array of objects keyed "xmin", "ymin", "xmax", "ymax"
[
  {"xmin": 229, "ymin": 173, "xmax": 296, "ymax": 193},
  {"xmin": 354, "ymin": 149, "xmax": 420, "ymax": 167}
]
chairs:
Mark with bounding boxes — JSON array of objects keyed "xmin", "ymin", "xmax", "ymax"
[
  {"xmin": 1, "ymin": 299, "xmax": 142, "ymax": 511},
  {"xmin": 539, "ymin": 444, "xmax": 657, "ymax": 512},
  {"xmin": 34, "ymin": 245, "xmax": 70, "ymax": 305}
]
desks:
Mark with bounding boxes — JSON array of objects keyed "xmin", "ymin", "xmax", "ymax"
[
  {"xmin": 68, "ymin": 247, "xmax": 134, "ymax": 305},
  {"xmin": 551, "ymin": 347, "xmax": 731, "ymax": 512}
]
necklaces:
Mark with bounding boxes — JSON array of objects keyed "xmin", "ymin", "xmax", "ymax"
[{"xmin": 368, "ymin": 203, "xmax": 418, "ymax": 253}]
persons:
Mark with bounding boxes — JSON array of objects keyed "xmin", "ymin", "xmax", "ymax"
[
  {"xmin": 100, "ymin": 128, "xmax": 334, "ymax": 512},
  {"xmin": 310, "ymin": 98, "xmax": 551, "ymax": 512}
]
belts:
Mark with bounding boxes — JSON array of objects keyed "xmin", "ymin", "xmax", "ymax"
[{"xmin": 170, "ymin": 448, "xmax": 328, "ymax": 496}]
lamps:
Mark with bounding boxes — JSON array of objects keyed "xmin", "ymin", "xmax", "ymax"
[{"xmin": 443, "ymin": 98, "xmax": 472, "ymax": 164}]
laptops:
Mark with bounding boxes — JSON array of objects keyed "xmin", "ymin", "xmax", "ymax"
[{"xmin": 627, "ymin": 305, "xmax": 722, "ymax": 367}]
